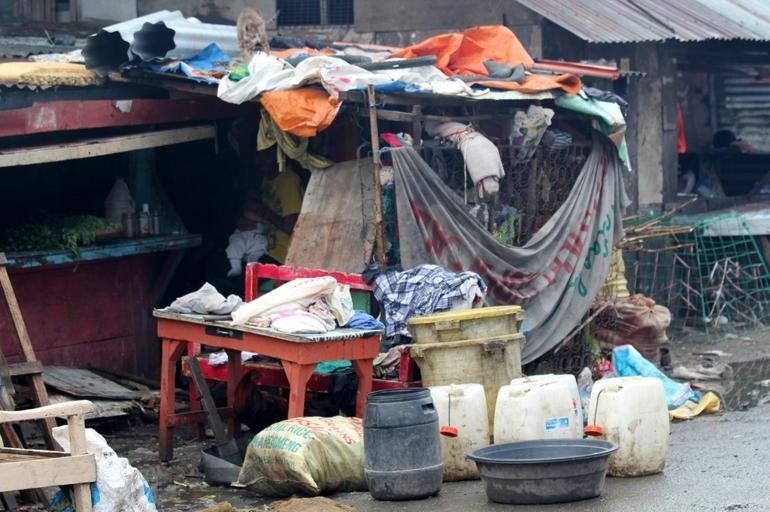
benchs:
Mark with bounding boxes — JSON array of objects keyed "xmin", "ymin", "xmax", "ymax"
[{"xmin": 182, "ymin": 262, "xmax": 422, "ymax": 440}]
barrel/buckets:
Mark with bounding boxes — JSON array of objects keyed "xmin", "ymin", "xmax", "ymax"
[
  {"xmin": 495, "ymin": 372, "xmax": 583, "ymax": 441},
  {"xmin": 408, "ymin": 306, "xmax": 523, "ymax": 339},
  {"xmin": 412, "ymin": 333, "xmax": 527, "ymax": 440},
  {"xmin": 432, "ymin": 384, "xmax": 490, "ymax": 479},
  {"xmin": 364, "ymin": 391, "xmax": 444, "ymax": 498},
  {"xmin": 587, "ymin": 375, "xmax": 668, "ymax": 476}
]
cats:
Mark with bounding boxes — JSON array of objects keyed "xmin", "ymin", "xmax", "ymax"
[{"xmin": 236, "ymin": 7, "xmax": 282, "ymax": 62}]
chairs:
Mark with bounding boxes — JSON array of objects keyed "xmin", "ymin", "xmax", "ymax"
[{"xmin": 0, "ymin": 400, "xmax": 96, "ymax": 512}]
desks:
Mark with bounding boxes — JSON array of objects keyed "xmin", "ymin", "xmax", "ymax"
[{"xmin": 152, "ymin": 308, "xmax": 385, "ymax": 462}]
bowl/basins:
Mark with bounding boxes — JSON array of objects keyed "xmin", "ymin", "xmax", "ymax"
[{"xmin": 466, "ymin": 438, "xmax": 619, "ymax": 504}]
